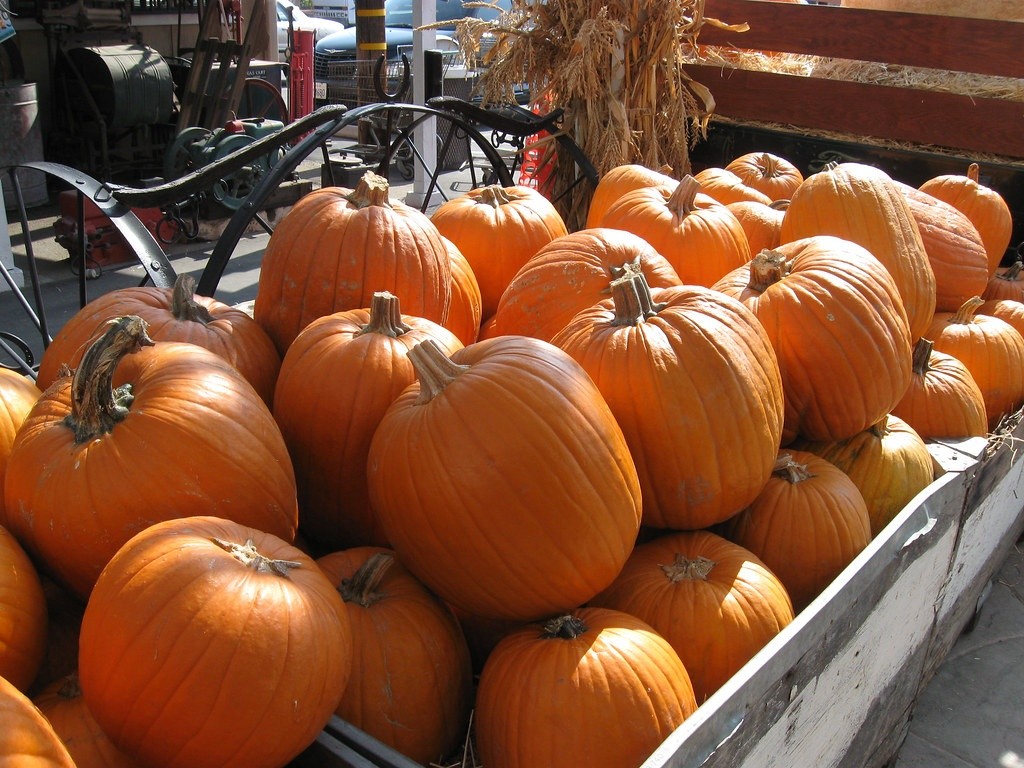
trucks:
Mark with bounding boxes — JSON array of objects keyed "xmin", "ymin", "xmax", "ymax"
[{"xmin": 34, "ymin": 0, "xmax": 1024, "ymax": 768}]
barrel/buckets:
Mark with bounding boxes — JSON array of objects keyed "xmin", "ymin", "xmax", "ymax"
[
  {"xmin": 0, "ymin": 81, "xmax": 49, "ymax": 211},
  {"xmin": 53, "ymin": 44, "xmax": 174, "ymax": 136}
]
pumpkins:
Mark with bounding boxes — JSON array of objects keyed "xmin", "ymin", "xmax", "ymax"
[{"xmin": 0, "ymin": 151, "xmax": 1023, "ymax": 768}]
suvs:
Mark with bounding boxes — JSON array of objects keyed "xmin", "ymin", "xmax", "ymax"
[
  {"xmin": 276, "ymin": 0, "xmax": 346, "ymax": 80},
  {"xmin": 313, "ymin": 0, "xmax": 550, "ymax": 126}
]
